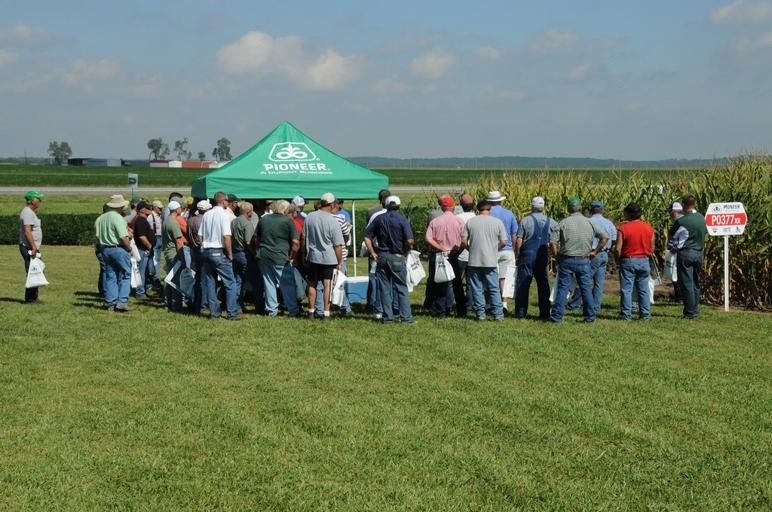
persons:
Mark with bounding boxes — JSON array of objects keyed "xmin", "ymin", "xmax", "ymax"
[
  {"xmin": 173, "ymin": 199, "xmax": 198, "ymax": 306},
  {"xmin": 338, "ymin": 200, "xmax": 351, "ymax": 276},
  {"xmin": 302, "ymin": 193, "xmax": 345, "ymax": 320},
  {"xmin": 666, "ymin": 202, "xmax": 685, "ymax": 306},
  {"xmin": 95, "ymin": 203, "xmax": 107, "ymax": 299},
  {"xmin": 365, "ymin": 197, "xmax": 388, "ymax": 321},
  {"xmin": 613, "ymin": 202, "xmax": 656, "ymax": 321},
  {"xmin": 461, "ymin": 200, "xmax": 506, "ymax": 321},
  {"xmin": 95, "ymin": 194, "xmax": 132, "ymax": 312},
  {"xmin": 484, "ymin": 191, "xmax": 518, "ymax": 315},
  {"xmin": 256, "ymin": 200, "xmax": 300, "ymax": 316},
  {"xmin": 231, "ymin": 202, "xmax": 256, "ymax": 306},
  {"xmin": 316, "ymin": 198, "xmax": 356, "ymax": 318},
  {"xmin": 565, "ymin": 201, "xmax": 617, "ymax": 310},
  {"xmin": 198, "ymin": 191, "xmax": 245, "ymax": 321},
  {"xmin": 18, "ymin": 190, "xmax": 44, "ymax": 303},
  {"xmin": 147, "ymin": 201, "xmax": 164, "ymax": 289},
  {"xmin": 454, "ymin": 194, "xmax": 478, "ymax": 317},
  {"xmin": 189, "ymin": 198, "xmax": 212, "ymax": 313},
  {"xmin": 364, "ymin": 195, "xmax": 415, "ymax": 324},
  {"xmin": 550, "ymin": 196, "xmax": 610, "ymax": 322},
  {"xmin": 428, "ymin": 194, "xmax": 452, "ymax": 313},
  {"xmin": 366, "ymin": 190, "xmax": 391, "ymax": 313},
  {"xmin": 162, "ymin": 201, "xmax": 186, "ymax": 312},
  {"xmin": 226, "ymin": 194, "xmax": 240, "ymax": 223},
  {"xmin": 133, "ymin": 201, "xmax": 156, "ymax": 299},
  {"xmin": 287, "ymin": 195, "xmax": 306, "ymax": 266},
  {"xmin": 671, "ymin": 195, "xmax": 707, "ymax": 319},
  {"xmin": 261, "ymin": 199, "xmax": 275, "ymax": 217},
  {"xmin": 516, "ymin": 196, "xmax": 559, "ymax": 320},
  {"xmin": 423, "ymin": 198, "xmax": 466, "ymax": 315}
]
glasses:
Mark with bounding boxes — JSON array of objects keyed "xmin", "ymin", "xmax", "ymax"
[{"xmin": 224, "ymin": 199, "xmax": 228, "ymax": 201}]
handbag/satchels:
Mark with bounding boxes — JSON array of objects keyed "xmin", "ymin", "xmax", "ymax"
[
  {"xmin": 151, "ymin": 213, "xmax": 157, "ymax": 234},
  {"xmin": 406, "ymin": 250, "xmax": 426, "ymax": 293},
  {"xmin": 549, "ymin": 264, "xmax": 570, "ymax": 304},
  {"xmin": 330, "ymin": 269, "xmax": 348, "ymax": 307},
  {"xmin": 502, "ymin": 265, "xmax": 518, "ymax": 299},
  {"xmin": 25, "ymin": 257, "xmax": 49, "ymax": 288},
  {"xmin": 179, "ymin": 267, "xmax": 196, "ymax": 297},
  {"xmin": 434, "ymin": 252, "xmax": 456, "ymax": 283},
  {"xmin": 130, "ymin": 257, "xmax": 143, "ymax": 288},
  {"xmin": 662, "ymin": 249, "xmax": 678, "ymax": 282}
]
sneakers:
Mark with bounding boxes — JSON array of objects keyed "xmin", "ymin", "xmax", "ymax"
[
  {"xmin": 308, "ymin": 313, "xmax": 314, "ymax": 318},
  {"xmin": 382, "ymin": 319, "xmax": 393, "ymax": 324},
  {"xmin": 147, "ymin": 288, "xmax": 160, "ymax": 297},
  {"xmin": 323, "ymin": 316, "xmax": 330, "ymax": 320},
  {"xmin": 550, "ymin": 314, "xmax": 561, "ymax": 322},
  {"xmin": 373, "ymin": 313, "xmax": 381, "ymax": 319},
  {"xmin": 683, "ymin": 310, "xmax": 698, "ymax": 319},
  {"xmin": 116, "ymin": 302, "xmax": 129, "ymax": 311},
  {"xmin": 477, "ymin": 315, "xmax": 486, "ymax": 321},
  {"xmin": 495, "ymin": 314, "xmax": 504, "ymax": 320},
  {"xmin": 107, "ymin": 305, "xmax": 115, "ymax": 311},
  {"xmin": 617, "ymin": 314, "xmax": 631, "ymax": 320},
  {"xmin": 584, "ymin": 317, "xmax": 595, "ymax": 323},
  {"xmin": 503, "ymin": 302, "xmax": 508, "ymax": 312},
  {"xmin": 640, "ymin": 314, "xmax": 649, "ymax": 320},
  {"xmin": 137, "ymin": 295, "xmax": 150, "ymax": 300},
  {"xmin": 401, "ymin": 320, "xmax": 417, "ymax": 325}
]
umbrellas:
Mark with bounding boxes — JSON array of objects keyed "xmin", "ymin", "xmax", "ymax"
[{"xmin": 192, "ymin": 122, "xmax": 390, "ymax": 199}]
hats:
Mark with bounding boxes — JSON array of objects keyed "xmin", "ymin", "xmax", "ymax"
[
  {"xmin": 292, "ymin": 196, "xmax": 305, "ymax": 206},
  {"xmin": 385, "ymin": 196, "xmax": 401, "ymax": 207},
  {"xmin": 485, "ymin": 191, "xmax": 506, "ymax": 202},
  {"xmin": 531, "ymin": 197, "xmax": 544, "ymax": 209},
  {"xmin": 568, "ymin": 197, "xmax": 581, "ymax": 209},
  {"xmin": 107, "ymin": 195, "xmax": 129, "ymax": 208},
  {"xmin": 667, "ymin": 202, "xmax": 683, "ymax": 211},
  {"xmin": 441, "ymin": 198, "xmax": 455, "ymax": 207},
  {"xmin": 168, "ymin": 201, "xmax": 181, "ymax": 211},
  {"xmin": 461, "ymin": 195, "xmax": 472, "ymax": 205},
  {"xmin": 590, "ymin": 201, "xmax": 603, "ymax": 208},
  {"xmin": 322, "ymin": 193, "xmax": 335, "ymax": 207},
  {"xmin": 138, "ymin": 201, "xmax": 152, "ymax": 209},
  {"xmin": 228, "ymin": 194, "xmax": 240, "ymax": 202},
  {"xmin": 153, "ymin": 200, "xmax": 164, "ymax": 208},
  {"xmin": 25, "ymin": 190, "xmax": 44, "ymax": 200},
  {"xmin": 186, "ymin": 197, "xmax": 194, "ymax": 205},
  {"xmin": 197, "ymin": 200, "xmax": 212, "ymax": 210},
  {"xmin": 477, "ymin": 200, "xmax": 490, "ymax": 210}
]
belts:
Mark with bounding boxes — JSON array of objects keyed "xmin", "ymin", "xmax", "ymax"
[
  {"xmin": 621, "ymin": 255, "xmax": 648, "ymax": 258},
  {"xmin": 564, "ymin": 256, "xmax": 589, "ymax": 259},
  {"xmin": 599, "ymin": 249, "xmax": 607, "ymax": 252},
  {"xmin": 104, "ymin": 245, "xmax": 122, "ymax": 247}
]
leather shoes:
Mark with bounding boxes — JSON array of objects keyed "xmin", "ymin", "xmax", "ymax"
[
  {"xmin": 230, "ymin": 316, "xmax": 247, "ymax": 321},
  {"xmin": 211, "ymin": 315, "xmax": 225, "ymax": 319},
  {"xmin": 26, "ymin": 299, "xmax": 36, "ymax": 304},
  {"xmin": 36, "ymin": 298, "xmax": 40, "ymax": 303}
]
